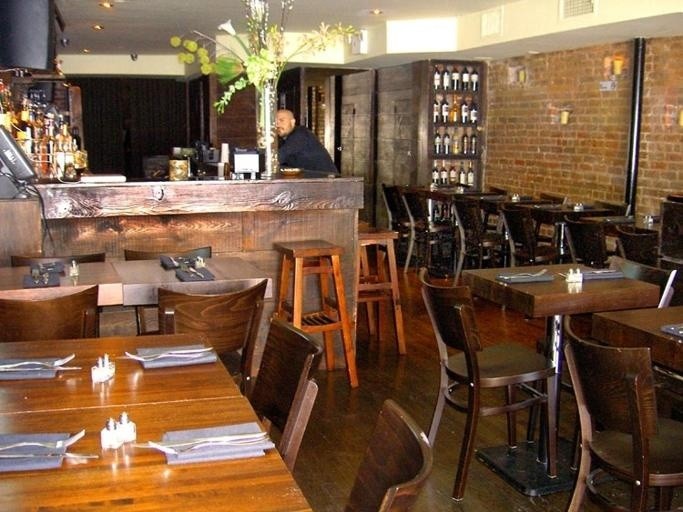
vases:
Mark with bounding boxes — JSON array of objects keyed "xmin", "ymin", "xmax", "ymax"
[{"xmin": 254, "ymin": 86, "xmax": 279, "ymax": 181}]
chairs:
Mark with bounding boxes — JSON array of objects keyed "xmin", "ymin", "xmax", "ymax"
[
  {"xmin": 500, "ymin": 206, "xmax": 554, "ymax": 268},
  {"xmin": 482, "ymin": 186, "xmax": 507, "ymax": 233},
  {"xmin": 400, "ymin": 190, "xmax": 452, "ymax": 274},
  {"xmin": 615, "ymin": 223, "xmax": 663, "ymax": 268},
  {"xmin": 0, "ymin": 284, "xmax": 98, "ymax": 343},
  {"xmin": 526, "ymin": 256, "xmax": 676, "ymax": 471},
  {"xmin": 417, "ymin": 267, "xmax": 558, "ymax": 504},
  {"xmin": 563, "ymin": 214, "xmax": 609, "ymax": 269},
  {"xmin": 593, "ymin": 200, "xmax": 626, "ymax": 216},
  {"xmin": 249, "ymin": 318, "xmax": 323, "ymax": 474},
  {"xmin": 380, "ymin": 183, "xmax": 423, "ymax": 261},
  {"xmin": 10, "ymin": 252, "xmax": 105, "ymax": 312},
  {"xmin": 134, "ymin": 277, "xmax": 268, "ymax": 397},
  {"xmin": 563, "ymin": 314, "xmax": 683, "ymax": 512},
  {"xmin": 123, "ymin": 246, "xmax": 211, "ymax": 336},
  {"xmin": 455, "ymin": 182, "xmax": 470, "ymax": 191},
  {"xmin": 451, "ymin": 200, "xmax": 500, "ymax": 287},
  {"xmin": 535, "ymin": 192, "xmax": 567, "ymax": 243},
  {"xmin": 340, "ymin": 398, "xmax": 433, "ymax": 512}
]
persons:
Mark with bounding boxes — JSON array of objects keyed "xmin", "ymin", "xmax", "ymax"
[{"xmin": 275, "ymin": 109, "xmax": 338, "ymax": 174}]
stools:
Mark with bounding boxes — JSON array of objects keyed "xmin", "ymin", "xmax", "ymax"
[
  {"xmin": 357, "ymin": 232, "xmax": 405, "ymax": 356},
  {"xmin": 357, "ymin": 221, "xmax": 374, "ymax": 335},
  {"xmin": 273, "ymin": 240, "xmax": 359, "ymax": 390}
]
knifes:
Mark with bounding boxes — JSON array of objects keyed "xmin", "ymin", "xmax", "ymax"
[
  {"xmin": 129, "ymin": 431, "xmax": 267, "ymax": 448},
  {"xmin": 115, "ymin": 347, "xmax": 214, "ymax": 360},
  {"xmin": 189, "ymin": 267, "xmax": 204, "ymax": 278},
  {"xmin": 591, "ymin": 269, "xmax": 617, "ymax": 274},
  {"xmin": 0, "ymin": 452, "xmax": 100, "ymax": 460},
  {"xmin": 0, "ymin": 367, "xmax": 82, "ymax": 371}
]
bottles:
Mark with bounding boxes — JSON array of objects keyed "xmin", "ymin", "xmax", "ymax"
[
  {"xmin": 449, "ymin": 159, "xmax": 456, "ymax": 183},
  {"xmin": 443, "ymin": 128, "xmax": 450, "ymax": 154},
  {"xmin": 452, "ymin": 128, "xmax": 459, "ymax": 155},
  {"xmin": 471, "ymin": 126, "xmax": 478, "ymax": 154},
  {"xmin": 432, "ymin": 160, "xmax": 439, "ymax": 183},
  {"xmin": 566, "ymin": 268, "xmax": 575, "ymax": 282},
  {"xmin": 441, "ymin": 93, "xmax": 449, "ymax": 123},
  {"xmin": 1, "ymin": 78, "xmax": 82, "ymax": 182},
  {"xmin": 441, "ymin": 202, "xmax": 448, "ymax": 218},
  {"xmin": 462, "ymin": 128, "xmax": 469, "ymax": 154},
  {"xmin": 470, "ymin": 96, "xmax": 478, "ymax": 124},
  {"xmin": 468, "ymin": 161, "xmax": 474, "ymax": 187},
  {"xmin": 432, "ymin": 201, "xmax": 439, "ymax": 222},
  {"xmin": 434, "ymin": 95, "xmax": 440, "ymax": 123},
  {"xmin": 461, "ymin": 66, "xmax": 469, "ymax": 91},
  {"xmin": 471, "ymin": 66, "xmax": 479, "ymax": 92},
  {"xmin": 511, "ymin": 194, "xmax": 517, "ymax": 201},
  {"xmin": 575, "ymin": 268, "xmax": 583, "ymax": 282},
  {"xmin": 461, "ymin": 96, "xmax": 469, "ymax": 123},
  {"xmin": 580, "ymin": 203, "xmax": 583, "ymax": 211},
  {"xmin": 517, "ymin": 193, "xmax": 520, "ymax": 201},
  {"xmin": 104, "ymin": 354, "xmax": 115, "ymax": 378},
  {"xmin": 451, "ymin": 65, "xmax": 459, "ymax": 90},
  {"xmin": 434, "ymin": 126, "xmax": 442, "ymax": 154},
  {"xmin": 433, "ymin": 66, "xmax": 441, "ymax": 90},
  {"xmin": 649, "ymin": 216, "xmax": 653, "ymax": 223},
  {"xmin": 574, "ymin": 203, "xmax": 579, "ymax": 211},
  {"xmin": 442, "ymin": 64, "xmax": 450, "ymax": 90},
  {"xmin": 118, "ymin": 415, "xmax": 136, "ymax": 442},
  {"xmin": 450, "ymin": 95, "xmax": 460, "ymax": 122},
  {"xmin": 91, "ymin": 357, "xmax": 107, "ymax": 383},
  {"xmin": 101, "ymin": 418, "xmax": 122, "ymax": 449},
  {"xmin": 440, "ymin": 159, "xmax": 448, "ymax": 184},
  {"xmin": 459, "ymin": 162, "xmax": 466, "ymax": 188},
  {"xmin": 642, "ymin": 216, "xmax": 648, "ymax": 224}
]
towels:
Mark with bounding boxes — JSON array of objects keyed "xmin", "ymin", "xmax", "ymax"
[
  {"xmin": 0, "ymin": 432, "xmax": 70, "ymax": 472},
  {"xmin": 23, "ymin": 270, "xmax": 60, "ymax": 289},
  {"xmin": 176, "ymin": 266, "xmax": 215, "ymax": 282},
  {"xmin": 496, "ymin": 272, "xmax": 554, "ymax": 283},
  {"xmin": 0, "ymin": 357, "xmax": 59, "ymax": 380},
  {"xmin": 31, "ymin": 261, "xmax": 66, "ymax": 273},
  {"xmin": 164, "ymin": 422, "xmax": 275, "ymax": 465},
  {"xmin": 662, "ymin": 323, "xmax": 683, "ymax": 339},
  {"xmin": 562, "ymin": 269, "xmax": 624, "ymax": 279},
  {"xmin": 160, "ymin": 255, "xmax": 195, "ymax": 268},
  {"xmin": 136, "ymin": 344, "xmax": 218, "ymax": 369}
]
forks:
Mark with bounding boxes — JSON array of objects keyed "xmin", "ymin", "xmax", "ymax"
[
  {"xmin": 181, "ymin": 262, "xmax": 195, "ymax": 279},
  {"xmin": 499, "ymin": 269, "xmax": 547, "ymax": 279},
  {"xmin": 0, "ymin": 353, "xmax": 76, "ymax": 368},
  {"xmin": 147, "ymin": 436, "xmax": 270, "ymax": 455},
  {"xmin": 0, "ymin": 429, "xmax": 86, "ymax": 451},
  {"xmin": 124, "ymin": 351, "xmax": 211, "ymax": 362}
]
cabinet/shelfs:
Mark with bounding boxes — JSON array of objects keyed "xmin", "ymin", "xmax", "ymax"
[{"xmin": 428, "ymin": 59, "xmax": 488, "ymax": 245}]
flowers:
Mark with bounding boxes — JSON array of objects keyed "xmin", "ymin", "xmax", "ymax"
[{"xmin": 168, "ymin": 1, "xmax": 363, "ymax": 109}]
